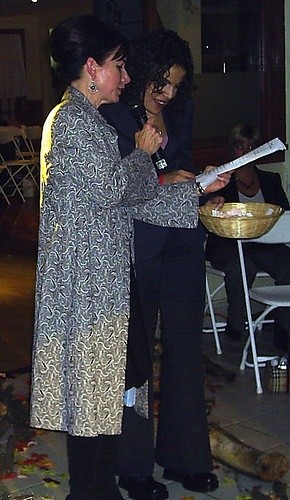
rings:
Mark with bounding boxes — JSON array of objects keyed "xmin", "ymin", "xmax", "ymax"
[{"xmin": 158, "ymin": 131, "xmax": 163, "ymax": 135}]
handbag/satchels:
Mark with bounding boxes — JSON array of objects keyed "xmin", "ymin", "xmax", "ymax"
[{"xmin": 264, "ymin": 360, "xmax": 287, "ymax": 393}]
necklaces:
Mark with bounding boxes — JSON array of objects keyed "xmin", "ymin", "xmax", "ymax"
[{"xmin": 236, "ymin": 171, "xmax": 258, "ymax": 189}]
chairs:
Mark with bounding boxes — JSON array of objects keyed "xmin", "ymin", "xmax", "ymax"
[
  {"xmin": 202, "ymin": 237, "xmax": 272, "ymax": 355},
  {"xmin": 238, "ymin": 210, "xmax": 290, "ymax": 395},
  {"xmin": 0, "ymin": 125, "xmax": 42, "ymax": 204}
]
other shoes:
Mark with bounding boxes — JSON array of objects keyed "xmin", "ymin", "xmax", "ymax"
[
  {"xmin": 119, "ymin": 476, "xmax": 168, "ymax": 500},
  {"xmin": 225, "ymin": 325, "xmax": 251, "ymax": 339},
  {"xmin": 162, "ymin": 471, "xmax": 219, "ymax": 492},
  {"xmin": 273, "ymin": 331, "xmax": 290, "ymax": 349}
]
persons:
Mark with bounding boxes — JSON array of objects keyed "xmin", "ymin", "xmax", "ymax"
[
  {"xmin": 205, "ymin": 123, "xmax": 289, "ymax": 373},
  {"xmin": 29, "ymin": 14, "xmax": 235, "ymax": 500}
]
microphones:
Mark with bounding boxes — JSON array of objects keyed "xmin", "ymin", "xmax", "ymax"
[{"xmin": 126, "ymin": 99, "xmax": 169, "ymax": 172}]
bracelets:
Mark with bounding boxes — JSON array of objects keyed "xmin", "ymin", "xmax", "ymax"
[{"xmin": 196, "ymin": 183, "xmax": 204, "ymax": 194}]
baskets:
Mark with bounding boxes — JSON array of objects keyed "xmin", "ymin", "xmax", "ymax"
[{"xmin": 197, "ymin": 202, "xmax": 285, "ymax": 239}]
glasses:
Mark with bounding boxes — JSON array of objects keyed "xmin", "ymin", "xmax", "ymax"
[{"xmin": 232, "ymin": 143, "xmax": 254, "ymax": 151}]
